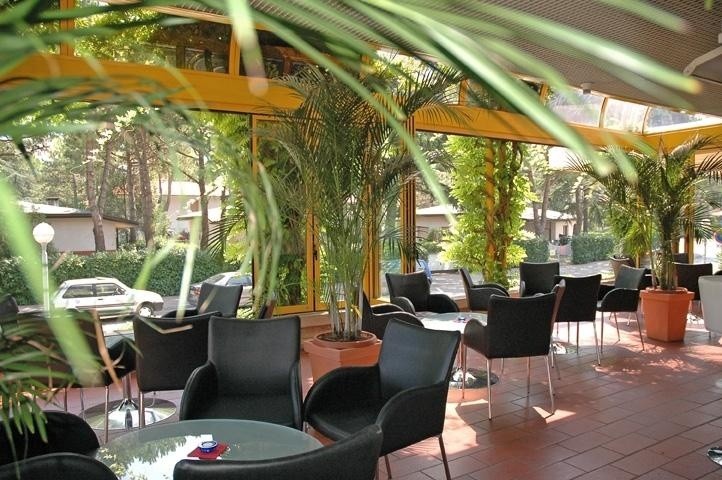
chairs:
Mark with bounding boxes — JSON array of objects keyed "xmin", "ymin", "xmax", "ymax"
[
  {"xmin": 2, "ymin": 411, "xmax": 100, "ymax": 466},
  {"xmin": 1, "ymin": 452, "xmax": 117, "ymax": 479},
  {"xmin": 172, "ymin": 424, "xmax": 383, "ymax": 478},
  {"xmin": 179, "ymin": 316, "xmax": 303, "ymax": 431},
  {"xmin": 353, "ymin": 262, "xmax": 566, "ymax": 420},
  {"xmin": 2, "ymin": 282, "xmax": 244, "ymax": 444},
  {"xmin": 552, "ymin": 251, "xmax": 721, "ymax": 370},
  {"xmin": 302, "ymin": 318, "xmax": 461, "ymax": 478}
]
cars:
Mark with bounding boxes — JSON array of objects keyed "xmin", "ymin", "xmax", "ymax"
[
  {"xmin": 187, "ymin": 271, "xmax": 252, "ymax": 310},
  {"xmin": 373, "ymin": 259, "xmax": 432, "ymax": 298},
  {"xmin": 18, "ymin": 276, "xmax": 167, "ymax": 320}
]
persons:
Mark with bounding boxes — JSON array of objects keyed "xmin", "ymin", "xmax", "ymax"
[{"xmin": 178, "ymin": 228, "xmax": 190, "ymax": 241}]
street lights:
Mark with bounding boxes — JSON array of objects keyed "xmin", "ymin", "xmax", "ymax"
[{"xmin": 33, "ymin": 220, "xmax": 55, "ymax": 318}]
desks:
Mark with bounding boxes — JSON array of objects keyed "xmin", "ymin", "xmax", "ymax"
[{"xmin": 86, "ymin": 419, "xmax": 325, "ymax": 479}]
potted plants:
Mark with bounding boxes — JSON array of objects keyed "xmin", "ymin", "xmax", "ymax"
[
  {"xmin": 569, "ymin": 129, "xmax": 722, "ymax": 342},
  {"xmin": 247, "ymin": 51, "xmax": 481, "ymax": 390}
]
